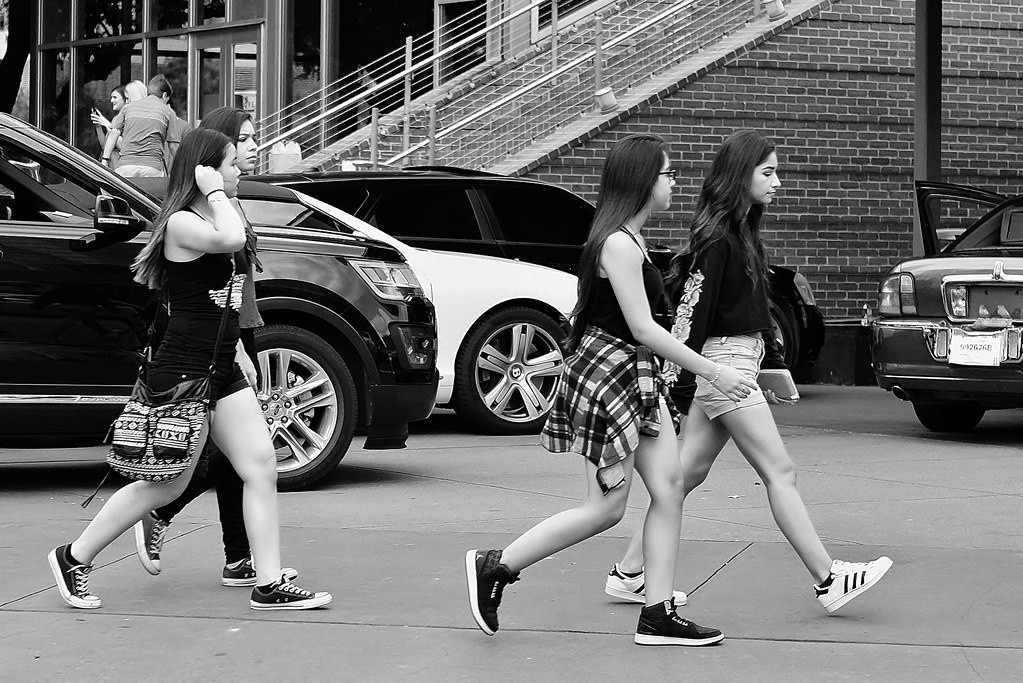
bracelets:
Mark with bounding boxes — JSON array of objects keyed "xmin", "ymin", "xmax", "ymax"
[
  {"xmin": 707, "ymin": 363, "xmax": 721, "ymax": 382},
  {"xmin": 102, "ymin": 156, "xmax": 111, "ymax": 162},
  {"xmin": 209, "ymin": 196, "xmax": 229, "ymax": 206},
  {"xmin": 206, "ymin": 190, "xmax": 225, "ymax": 199}
]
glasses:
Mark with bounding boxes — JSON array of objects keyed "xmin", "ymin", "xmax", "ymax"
[{"xmin": 660, "ymin": 167, "xmax": 679, "ymax": 182}]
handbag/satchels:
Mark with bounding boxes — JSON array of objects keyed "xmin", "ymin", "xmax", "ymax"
[{"xmin": 106, "ymin": 375, "xmax": 212, "ymax": 485}]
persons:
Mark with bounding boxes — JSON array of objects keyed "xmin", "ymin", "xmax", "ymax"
[
  {"xmin": 90, "ymin": 75, "xmax": 190, "ymax": 178},
  {"xmin": 464, "ymin": 133, "xmax": 758, "ymax": 646},
  {"xmin": 134, "ymin": 107, "xmax": 297, "ymax": 587},
  {"xmin": 605, "ymin": 128, "xmax": 895, "ymax": 614},
  {"xmin": 47, "ymin": 127, "xmax": 332, "ymax": 610}
]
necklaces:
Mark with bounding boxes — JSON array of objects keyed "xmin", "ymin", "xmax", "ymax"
[{"xmin": 190, "ymin": 202, "xmax": 214, "ymax": 224}]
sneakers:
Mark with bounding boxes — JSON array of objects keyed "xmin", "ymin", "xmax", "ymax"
[
  {"xmin": 222, "ymin": 559, "xmax": 299, "ymax": 586},
  {"xmin": 48, "ymin": 544, "xmax": 102, "ymax": 609},
  {"xmin": 605, "ymin": 562, "xmax": 688, "ymax": 606},
  {"xmin": 250, "ymin": 573, "xmax": 332, "ymax": 611},
  {"xmin": 635, "ymin": 596, "xmax": 725, "ymax": 646},
  {"xmin": 135, "ymin": 514, "xmax": 172, "ymax": 575},
  {"xmin": 813, "ymin": 555, "xmax": 894, "ymax": 614},
  {"xmin": 464, "ymin": 548, "xmax": 519, "ymax": 636}
]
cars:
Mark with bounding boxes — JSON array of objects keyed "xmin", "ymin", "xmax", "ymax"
[
  {"xmin": 40, "ymin": 172, "xmax": 581, "ymax": 436},
  {"xmin": 236, "ymin": 166, "xmax": 677, "ymax": 341},
  {"xmin": 642, "ymin": 239, "xmax": 828, "ymax": 380},
  {"xmin": 0, "ymin": 112, "xmax": 446, "ymax": 489},
  {"xmin": 870, "ymin": 181, "xmax": 1022, "ymax": 437}
]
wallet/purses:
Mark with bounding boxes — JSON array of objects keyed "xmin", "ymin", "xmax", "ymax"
[{"xmin": 756, "ymin": 368, "xmax": 798, "ymax": 402}]
